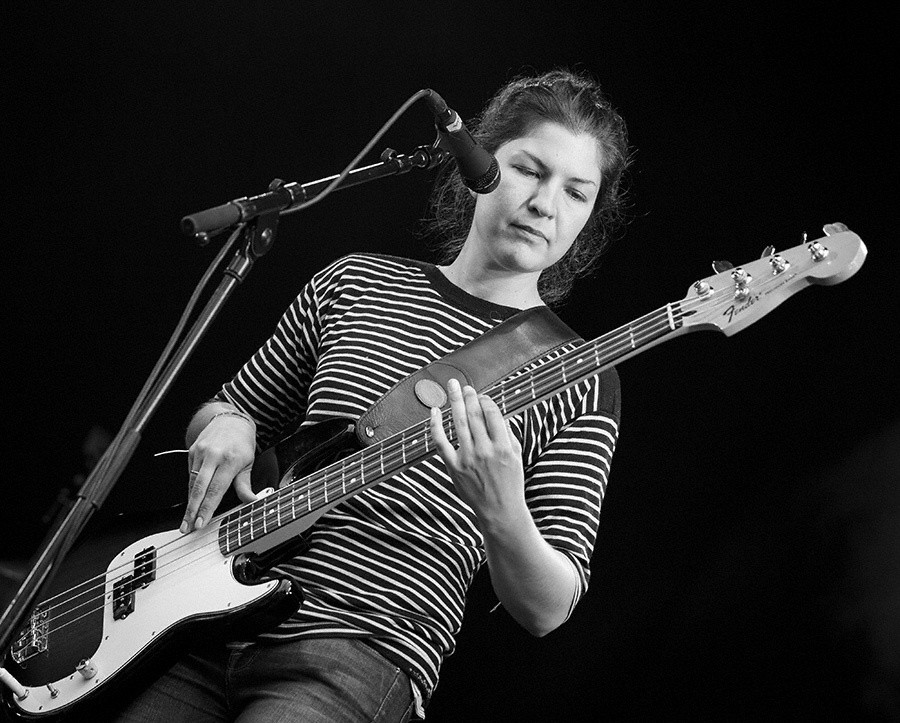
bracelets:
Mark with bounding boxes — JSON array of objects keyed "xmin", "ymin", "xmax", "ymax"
[{"xmin": 209, "ymin": 410, "xmax": 256, "ymax": 433}]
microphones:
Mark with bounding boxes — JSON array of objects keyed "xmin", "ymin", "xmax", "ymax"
[{"xmin": 427, "ymin": 89, "xmax": 502, "ymax": 195}]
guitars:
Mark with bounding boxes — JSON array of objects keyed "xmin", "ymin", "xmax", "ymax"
[{"xmin": 0, "ymin": 221, "xmax": 870, "ymax": 717}]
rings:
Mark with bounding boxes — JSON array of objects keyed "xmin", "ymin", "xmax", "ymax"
[{"xmin": 189, "ymin": 469, "xmax": 199, "ymax": 475}]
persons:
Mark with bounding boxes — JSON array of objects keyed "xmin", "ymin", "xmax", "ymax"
[{"xmin": 113, "ymin": 71, "xmax": 633, "ymax": 723}]
